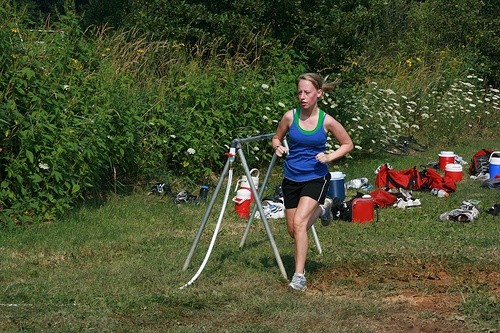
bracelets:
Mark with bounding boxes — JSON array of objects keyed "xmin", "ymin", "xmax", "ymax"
[{"xmin": 274, "ymin": 146, "xmax": 279, "ymax": 150}]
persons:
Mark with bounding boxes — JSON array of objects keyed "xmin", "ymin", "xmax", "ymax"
[{"xmin": 272, "ymin": 73, "xmax": 354, "ymax": 294}]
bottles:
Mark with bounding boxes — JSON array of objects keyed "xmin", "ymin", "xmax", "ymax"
[{"xmin": 200, "ymin": 185, "xmax": 208, "ymax": 202}]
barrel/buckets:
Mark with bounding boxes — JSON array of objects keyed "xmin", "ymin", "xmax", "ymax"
[
  {"xmin": 234, "ymin": 168, "xmax": 259, "ymax": 209},
  {"xmin": 352, "ymin": 198, "xmax": 374, "ymax": 222},
  {"xmin": 489, "ymin": 151, "xmax": 500, "ymax": 180},
  {"xmin": 327, "ymin": 171, "xmax": 346, "ymax": 200},
  {"xmin": 231, "ymin": 190, "xmax": 251, "ymax": 219},
  {"xmin": 438, "ymin": 150, "xmax": 454, "ymax": 172},
  {"xmin": 444, "ymin": 164, "xmax": 462, "ymax": 181}
]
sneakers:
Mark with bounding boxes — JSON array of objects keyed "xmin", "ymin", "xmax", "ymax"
[
  {"xmin": 440, "ymin": 211, "xmax": 474, "ymax": 223},
  {"xmin": 392, "ymin": 197, "xmax": 421, "ymax": 208},
  {"xmin": 321, "ymin": 198, "xmax": 333, "ymax": 227},
  {"xmin": 289, "ymin": 273, "xmax": 307, "ymax": 292},
  {"xmin": 254, "ymin": 203, "xmax": 284, "ymax": 220}
]
